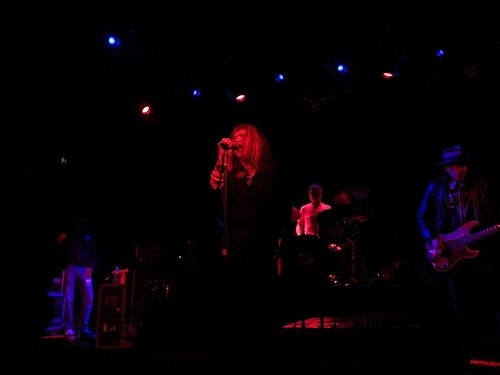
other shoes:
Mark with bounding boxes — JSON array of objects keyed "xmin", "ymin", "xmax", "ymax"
[
  {"xmin": 66, "ymin": 329, "xmax": 75, "ymax": 339},
  {"xmin": 83, "ymin": 327, "xmax": 95, "ymax": 340}
]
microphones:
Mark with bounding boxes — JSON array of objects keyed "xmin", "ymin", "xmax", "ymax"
[{"xmin": 220, "ymin": 143, "xmax": 238, "ymax": 150}]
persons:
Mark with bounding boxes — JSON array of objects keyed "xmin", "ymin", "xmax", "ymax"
[
  {"xmin": 294, "ymin": 184, "xmax": 333, "ymax": 240},
  {"xmin": 55, "ymin": 213, "xmax": 97, "ymax": 340},
  {"xmin": 208, "ymin": 123, "xmax": 280, "ymax": 268},
  {"xmin": 416, "ymin": 145, "xmax": 486, "ymax": 308}
]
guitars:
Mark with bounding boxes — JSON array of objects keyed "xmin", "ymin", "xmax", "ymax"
[{"xmin": 424, "ymin": 219, "xmax": 500, "ymax": 273}]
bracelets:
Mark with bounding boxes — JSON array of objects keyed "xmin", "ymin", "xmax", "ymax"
[
  {"xmin": 215, "ymin": 163, "xmax": 228, "ymax": 172},
  {"xmin": 209, "ymin": 173, "xmax": 225, "ymax": 183}
]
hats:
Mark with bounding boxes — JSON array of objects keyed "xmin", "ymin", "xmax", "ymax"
[{"xmin": 439, "ymin": 144, "xmax": 470, "ymax": 166}]
